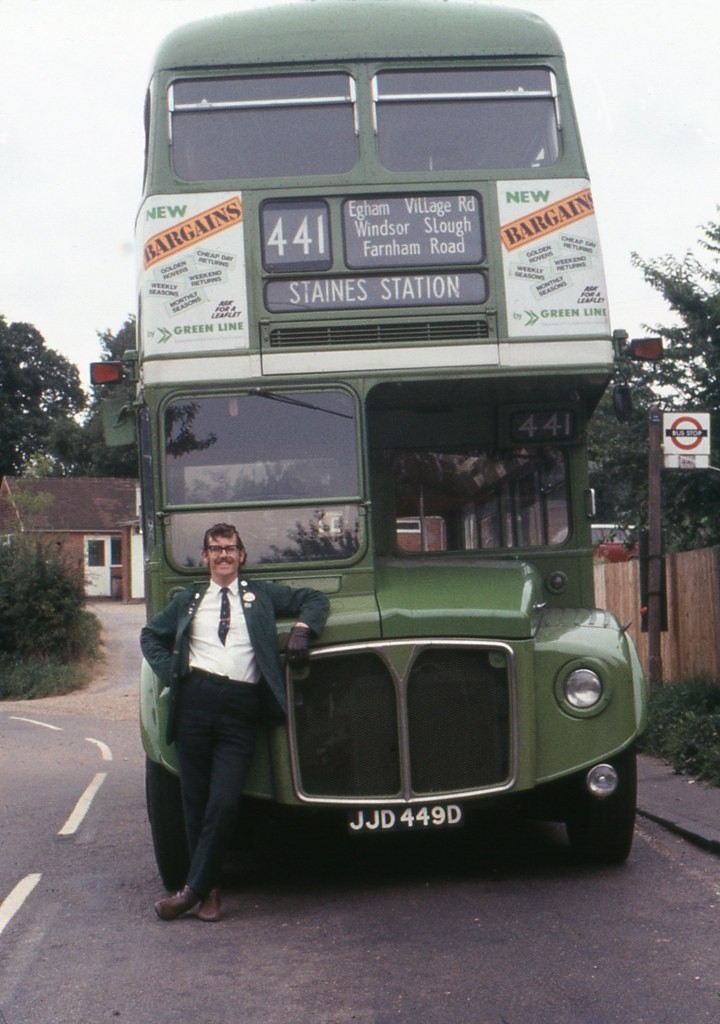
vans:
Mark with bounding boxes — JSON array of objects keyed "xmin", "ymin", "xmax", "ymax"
[{"xmin": 551, "ymin": 523, "xmax": 640, "ymax": 564}]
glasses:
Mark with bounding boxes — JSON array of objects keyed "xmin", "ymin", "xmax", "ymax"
[{"xmin": 207, "ymin": 545, "xmax": 239, "ymax": 554}]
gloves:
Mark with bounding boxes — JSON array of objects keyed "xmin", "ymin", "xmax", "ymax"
[{"xmin": 285, "ymin": 626, "xmax": 312, "ymax": 669}]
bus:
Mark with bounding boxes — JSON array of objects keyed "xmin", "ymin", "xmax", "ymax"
[{"xmin": 88, "ymin": 2, "xmax": 664, "ymax": 889}]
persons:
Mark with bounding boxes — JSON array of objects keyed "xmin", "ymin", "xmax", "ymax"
[{"xmin": 141, "ymin": 523, "xmax": 330, "ymax": 921}]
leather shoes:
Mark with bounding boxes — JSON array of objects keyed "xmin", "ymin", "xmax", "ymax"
[
  {"xmin": 153, "ymin": 884, "xmax": 200, "ymax": 919},
  {"xmin": 198, "ymin": 885, "xmax": 221, "ymax": 921}
]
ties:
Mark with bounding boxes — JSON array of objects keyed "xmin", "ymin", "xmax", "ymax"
[{"xmin": 218, "ymin": 588, "xmax": 230, "ymax": 646}]
